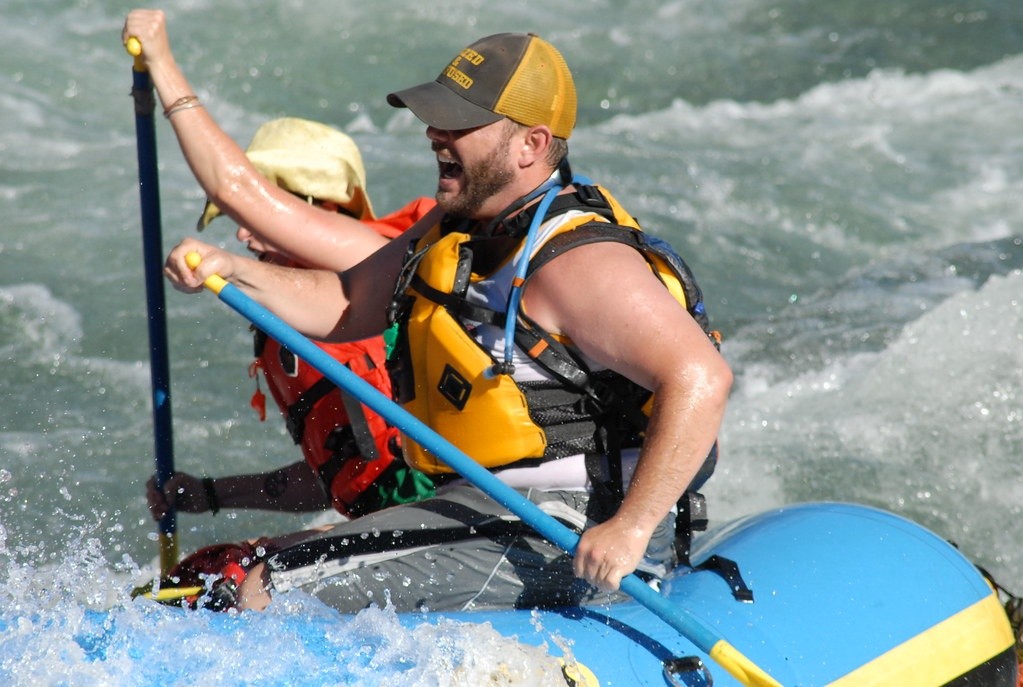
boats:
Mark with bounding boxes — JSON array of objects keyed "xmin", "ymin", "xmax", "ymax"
[{"xmin": 31, "ymin": 501, "xmax": 1023, "ymax": 687}]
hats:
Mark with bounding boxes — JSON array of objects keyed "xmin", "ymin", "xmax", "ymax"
[
  {"xmin": 387, "ymin": 33, "xmax": 577, "ymax": 141},
  {"xmin": 197, "ymin": 117, "xmax": 377, "ymax": 233}
]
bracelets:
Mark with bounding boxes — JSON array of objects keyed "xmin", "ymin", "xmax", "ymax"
[{"xmin": 165, "ymin": 95, "xmax": 200, "ymax": 116}]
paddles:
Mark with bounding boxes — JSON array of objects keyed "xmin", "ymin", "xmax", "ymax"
[
  {"xmin": 121, "ymin": 30, "xmax": 216, "ymax": 607},
  {"xmin": 182, "ymin": 245, "xmax": 791, "ymax": 687}
]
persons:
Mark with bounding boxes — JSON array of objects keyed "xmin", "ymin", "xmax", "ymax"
[
  {"xmin": 123, "ymin": 5, "xmax": 437, "ymax": 610},
  {"xmin": 164, "ymin": 32, "xmax": 734, "ymax": 616}
]
